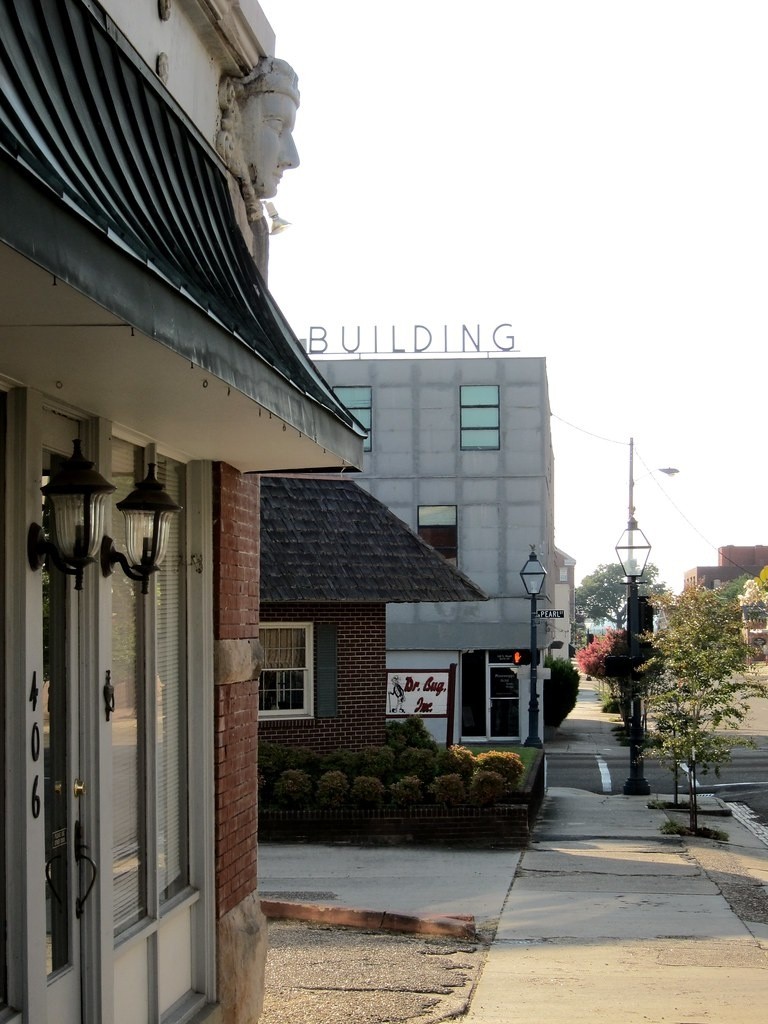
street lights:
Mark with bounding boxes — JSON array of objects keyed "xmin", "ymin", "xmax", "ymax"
[
  {"xmin": 584, "ymin": 617, "xmax": 593, "ymax": 681},
  {"xmin": 520, "ymin": 551, "xmax": 546, "ymax": 749},
  {"xmin": 615, "ymin": 520, "xmax": 652, "ymax": 795}
]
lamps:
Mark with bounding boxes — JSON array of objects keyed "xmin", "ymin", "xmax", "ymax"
[
  {"xmin": 261, "ymin": 201, "xmax": 292, "ymax": 235},
  {"xmin": 100, "ymin": 462, "xmax": 184, "ymax": 595},
  {"xmin": 27, "ymin": 438, "xmax": 117, "ymax": 591}
]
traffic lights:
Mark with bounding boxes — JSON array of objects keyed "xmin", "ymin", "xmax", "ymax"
[{"xmin": 514, "ymin": 648, "xmax": 530, "ymax": 666}]
